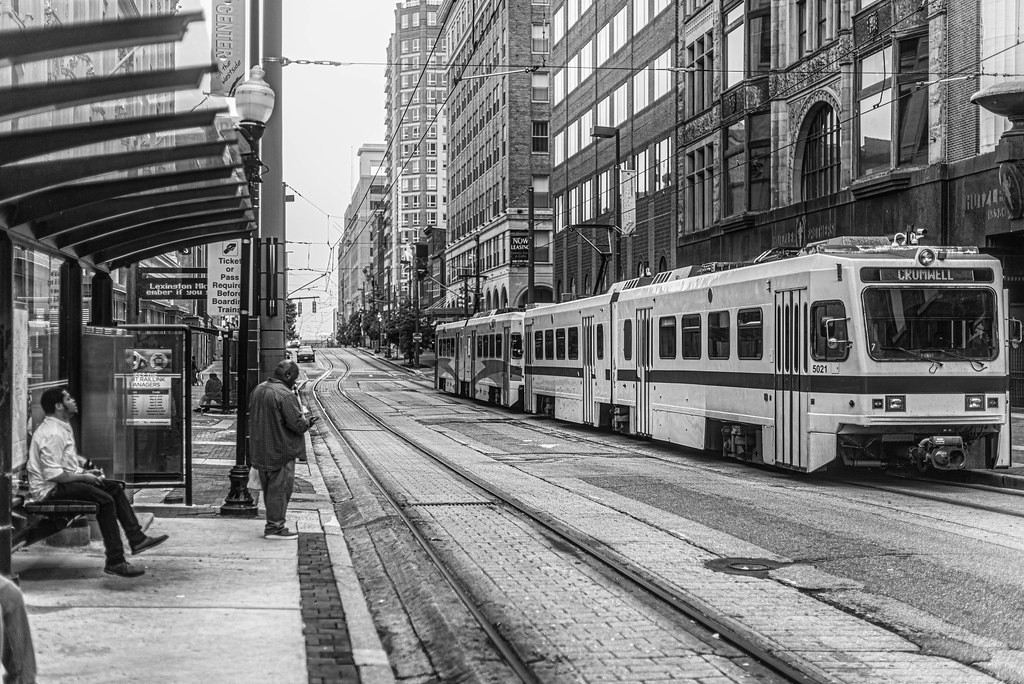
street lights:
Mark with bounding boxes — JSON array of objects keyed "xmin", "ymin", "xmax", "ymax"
[
  {"xmin": 218, "ymin": 62, "xmax": 275, "ymax": 515},
  {"xmin": 588, "ymin": 124, "xmax": 623, "ymax": 282}
]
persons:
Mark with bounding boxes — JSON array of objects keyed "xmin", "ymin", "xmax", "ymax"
[
  {"xmin": 193, "ymin": 373, "xmax": 226, "ymax": 414},
  {"xmin": 249, "ymin": 359, "xmax": 323, "ymax": 541},
  {"xmin": 31, "ymin": 384, "xmax": 170, "ymax": 577}
]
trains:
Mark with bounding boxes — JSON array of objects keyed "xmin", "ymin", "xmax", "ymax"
[{"xmin": 435, "ymin": 226, "xmax": 1014, "ymax": 484}]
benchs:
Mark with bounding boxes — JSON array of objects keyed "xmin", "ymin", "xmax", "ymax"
[{"xmin": 21, "ymin": 478, "xmax": 127, "ymax": 545}]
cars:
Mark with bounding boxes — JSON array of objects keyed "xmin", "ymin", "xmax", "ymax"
[
  {"xmin": 295, "ymin": 345, "xmax": 317, "ymax": 363},
  {"xmin": 327, "ymin": 337, "xmax": 341, "ymax": 348},
  {"xmin": 286, "ymin": 337, "xmax": 301, "ymax": 348}
]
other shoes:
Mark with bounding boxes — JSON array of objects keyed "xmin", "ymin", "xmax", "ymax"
[
  {"xmin": 264, "ymin": 528, "xmax": 299, "ymax": 539},
  {"xmin": 131, "ymin": 534, "xmax": 170, "ymax": 555},
  {"xmin": 103, "ymin": 562, "xmax": 146, "ymax": 579}
]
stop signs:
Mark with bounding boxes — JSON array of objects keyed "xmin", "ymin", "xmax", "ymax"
[{"xmin": 413, "ymin": 332, "xmax": 422, "ymax": 342}]
